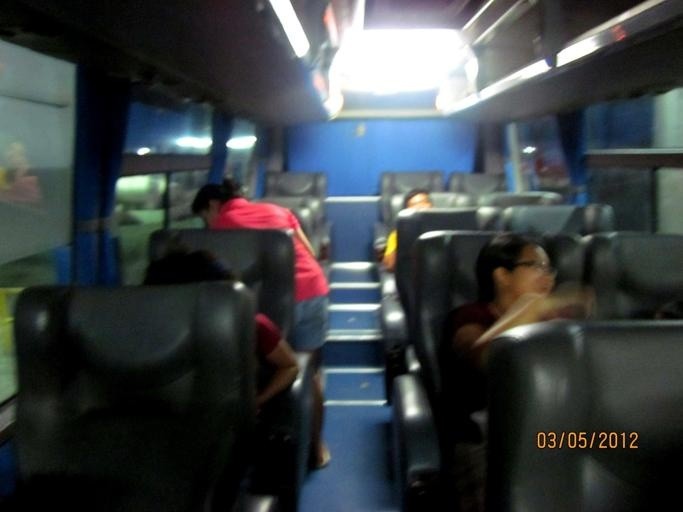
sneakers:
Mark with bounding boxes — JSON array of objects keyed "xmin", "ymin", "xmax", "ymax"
[{"xmin": 311, "ymin": 446, "xmax": 331, "ymax": 470}]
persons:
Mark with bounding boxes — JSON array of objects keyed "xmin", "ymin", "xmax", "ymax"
[
  {"xmin": 444, "ymin": 229, "xmax": 597, "ymax": 511},
  {"xmin": 0, "ymin": 137, "xmax": 34, "ymax": 194},
  {"xmin": 383, "ymin": 184, "xmax": 435, "ymax": 272},
  {"xmin": 189, "ymin": 178, "xmax": 333, "ymax": 470},
  {"xmin": 142, "ymin": 248, "xmax": 299, "ymax": 419}
]
